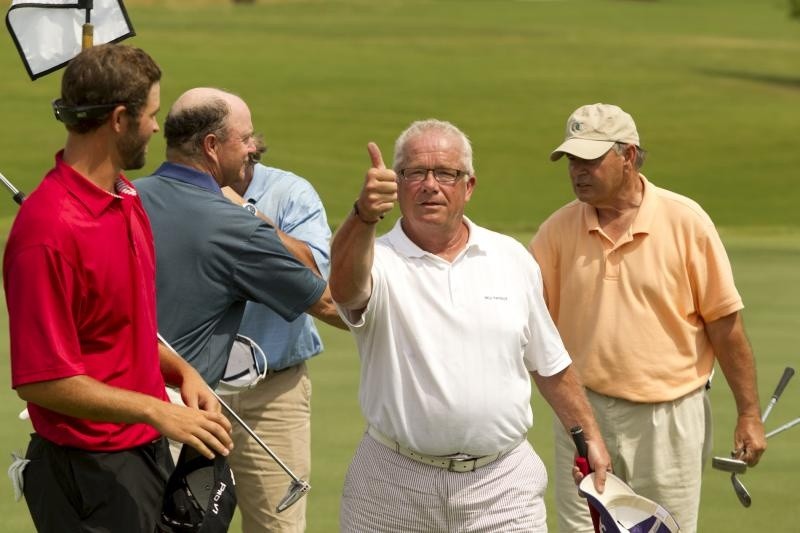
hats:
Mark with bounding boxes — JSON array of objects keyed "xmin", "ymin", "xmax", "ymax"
[
  {"xmin": 216, "ymin": 332, "xmax": 269, "ymax": 395},
  {"xmin": 579, "ymin": 469, "xmax": 680, "ymax": 533},
  {"xmin": 550, "ymin": 102, "xmax": 639, "ymax": 162},
  {"xmin": 159, "ymin": 441, "xmax": 236, "ymax": 533}
]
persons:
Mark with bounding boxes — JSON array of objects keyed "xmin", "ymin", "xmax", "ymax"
[
  {"xmin": 525, "ymin": 102, "xmax": 767, "ymax": 533},
  {"xmin": 134, "ymin": 86, "xmax": 358, "ymax": 533},
  {"xmin": 328, "ymin": 118, "xmax": 611, "ymax": 533},
  {"xmin": 3, "ymin": 43, "xmax": 237, "ymax": 533}
]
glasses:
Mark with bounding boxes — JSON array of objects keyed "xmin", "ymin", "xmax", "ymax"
[
  {"xmin": 393, "ymin": 167, "xmax": 470, "ymax": 183},
  {"xmin": 52, "ymin": 98, "xmax": 134, "ymax": 122}
]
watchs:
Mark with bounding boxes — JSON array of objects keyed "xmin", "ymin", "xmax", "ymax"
[
  {"xmin": 243, "ymin": 203, "xmax": 257, "ymax": 216},
  {"xmin": 352, "ymin": 201, "xmax": 379, "ymax": 225}
]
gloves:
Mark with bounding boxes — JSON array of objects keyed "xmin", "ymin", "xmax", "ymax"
[{"xmin": 8, "ymin": 452, "xmax": 30, "ymax": 499}]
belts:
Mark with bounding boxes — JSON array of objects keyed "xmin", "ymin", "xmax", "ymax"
[{"xmin": 367, "ymin": 424, "xmax": 526, "ymax": 473}]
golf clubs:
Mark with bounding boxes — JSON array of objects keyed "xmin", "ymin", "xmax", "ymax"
[
  {"xmin": 0, "ymin": 173, "xmax": 314, "ymax": 514},
  {"xmin": 570, "ymin": 425, "xmax": 602, "ymax": 533},
  {"xmin": 711, "ymin": 366, "xmax": 800, "ymax": 508}
]
